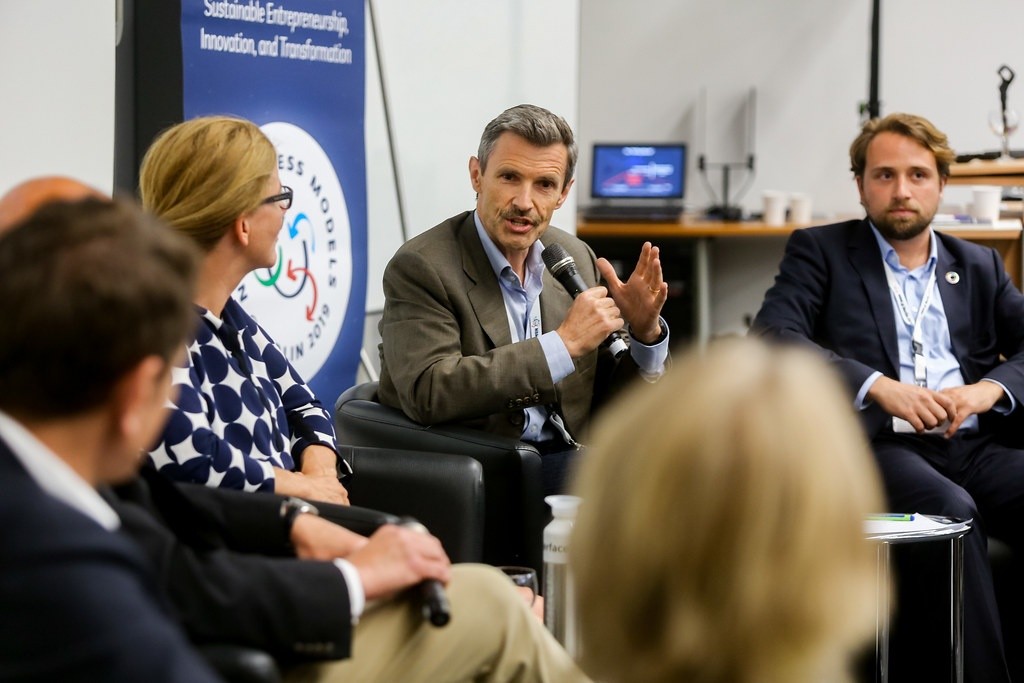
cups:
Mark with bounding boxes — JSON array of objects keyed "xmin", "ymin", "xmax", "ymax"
[
  {"xmin": 498, "ymin": 566, "xmax": 538, "ymax": 607},
  {"xmin": 972, "ymin": 189, "xmax": 1003, "ymax": 223},
  {"xmin": 789, "ymin": 196, "xmax": 814, "ymax": 224},
  {"xmin": 763, "ymin": 194, "xmax": 789, "ymax": 224}
]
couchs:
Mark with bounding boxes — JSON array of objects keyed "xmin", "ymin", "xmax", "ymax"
[{"xmin": 332, "ymin": 381, "xmax": 543, "ymax": 590}]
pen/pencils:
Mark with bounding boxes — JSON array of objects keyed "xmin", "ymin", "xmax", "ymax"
[
  {"xmin": 863, "ymin": 513, "xmax": 915, "ymax": 521},
  {"xmin": 931, "ymin": 214, "xmax": 992, "ymax": 226}
]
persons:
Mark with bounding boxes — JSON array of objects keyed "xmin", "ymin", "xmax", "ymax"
[
  {"xmin": 747, "ymin": 113, "xmax": 1024, "ymax": 683},
  {"xmin": 564, "ymin": 330, "xmax": 892, "ymax": 683},
  {"xmin": 377, "ymin": 104, "xmax": 672, "ymax": 489},
  {"xmin": 131, "ymin": 116, "xmax": 355, "ymax": 507},
  {"xmin": 0, "ymin": 196, "xmax": 593, "ymax": 683}
]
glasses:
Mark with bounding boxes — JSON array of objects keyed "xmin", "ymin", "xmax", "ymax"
[{"xmin": 260, "ymin": 186, "xmax": 294, "ymax": 209}]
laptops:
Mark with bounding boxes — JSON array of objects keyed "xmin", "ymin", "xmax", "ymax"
[{"xmin": 584, "ymin": 142, "xmax": 687, "ymax": 224}]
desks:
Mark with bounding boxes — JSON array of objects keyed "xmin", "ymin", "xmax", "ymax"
[
  {"xmin": 575, "ymin": 221, "xmax": 1024, "ymax": 335},
  {"xmin": 867, "ymin": 512, "xmax": 973, "ymax": 683}
]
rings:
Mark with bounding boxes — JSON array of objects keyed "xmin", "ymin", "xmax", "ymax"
[{"xmin": 649, "ymin": 286, "xmax": 660, "ymax": 293}]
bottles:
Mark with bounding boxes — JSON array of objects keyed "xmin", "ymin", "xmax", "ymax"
[{"xmin": 542, "ymin": 495, "xmax": 577, "ymax": 658}]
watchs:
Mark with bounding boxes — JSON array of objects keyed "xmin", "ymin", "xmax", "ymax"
[{"xmin": 284, "ymin": 496, "xmax": 320, "ymax": 534}]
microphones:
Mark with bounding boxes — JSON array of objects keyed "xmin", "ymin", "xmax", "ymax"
[
  {"xmin": 541, "ymin": 243, "xmax": 629, "ymax": 359},
  {"xmin": 397, "ymin": 517, "xmax": 451, "ymax": 627}
]
